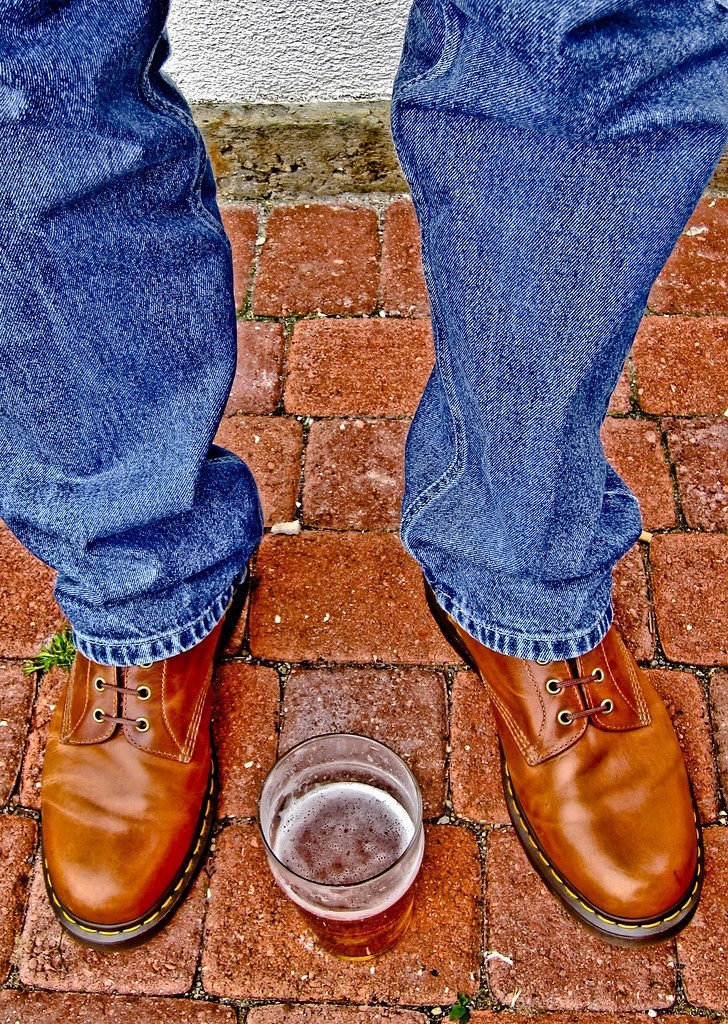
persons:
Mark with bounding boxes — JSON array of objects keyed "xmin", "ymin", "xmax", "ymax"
[{"xmin": 0, "ymin": 0, "xmax": 728, "ymax": 951}]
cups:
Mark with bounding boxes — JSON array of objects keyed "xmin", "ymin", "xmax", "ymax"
[{"xmin": 256, "ymin": 734, "xmax": 424, "ymax": 962}]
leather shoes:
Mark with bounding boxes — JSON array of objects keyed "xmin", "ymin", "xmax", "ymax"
[
  {"xmin": 38, "ymin": 575, "xmax": 253, "ymax": 948},
  {"xmin": 422, "ymin": 568, "xmax": 703, "ymax": 941}
]
